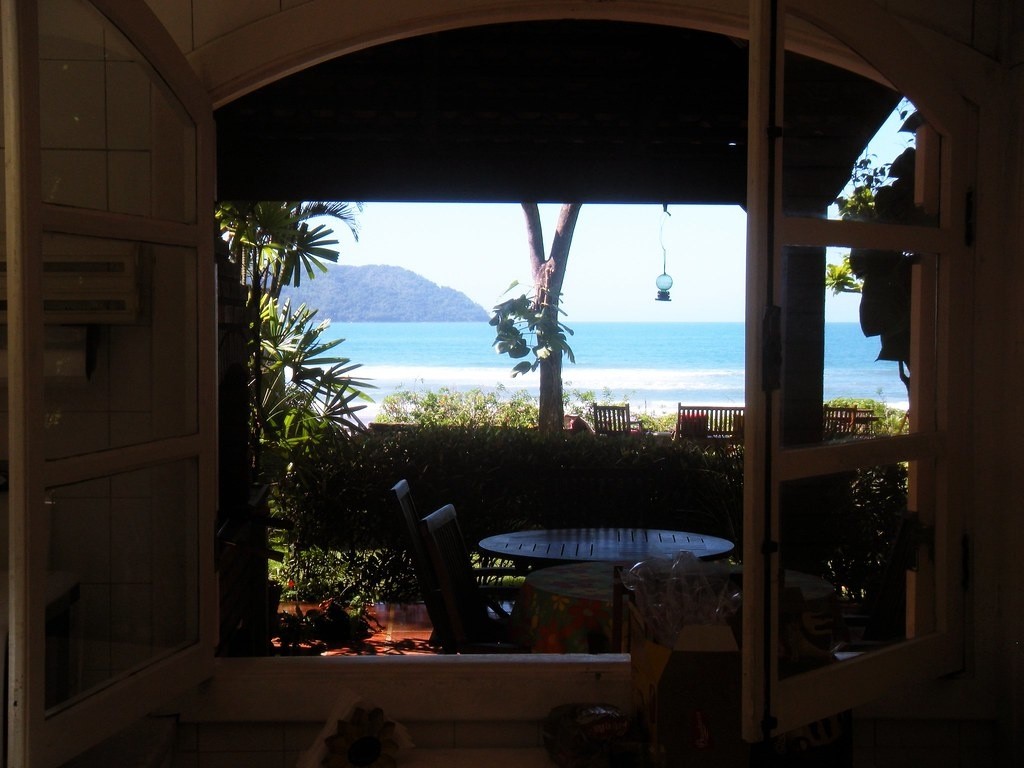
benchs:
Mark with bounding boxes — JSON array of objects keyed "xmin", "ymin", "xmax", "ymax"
[
  {"xmin": 677, "ymin": 402, "xmax": 745, "ymax": 447},
  {"xmin": 592, "ymin": 402, "xmax": 644, "ymax": 435},
  {"xmin": 822, "ymin": 403, "xmax": 879, "ymax": 433}
]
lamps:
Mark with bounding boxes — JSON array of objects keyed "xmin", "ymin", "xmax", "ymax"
[{"xmin": 655, "ymin": 210, "xmax": 673, "ymax": 302}]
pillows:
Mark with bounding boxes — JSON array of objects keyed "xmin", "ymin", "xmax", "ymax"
[
  {"xmin": 680, "ymin": 413, "xmax": 709, "ymax": 438},
  {"xmin": 732, "ymin": 412, "xmax": 744, "ymax": 436}
]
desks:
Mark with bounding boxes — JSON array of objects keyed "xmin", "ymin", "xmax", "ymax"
[
  {"xmin": 478, "ymin": 528, "xmax": 735, "ymax": 566},
  {"xmin": 503, "ymin": 561, "xmax": 838, "ymax": 654}
]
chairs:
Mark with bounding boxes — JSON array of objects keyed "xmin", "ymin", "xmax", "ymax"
[
  {"xmin": 391, "ymin": 479, "xmax": 531, "ymax": 654},
  {"xmin": 842, "ymin": 511, "xmax": 919, "ymax": 641},
  {"xmin": 610, "ymin": 565, "xmax": 742, "ymax": 654}
]
